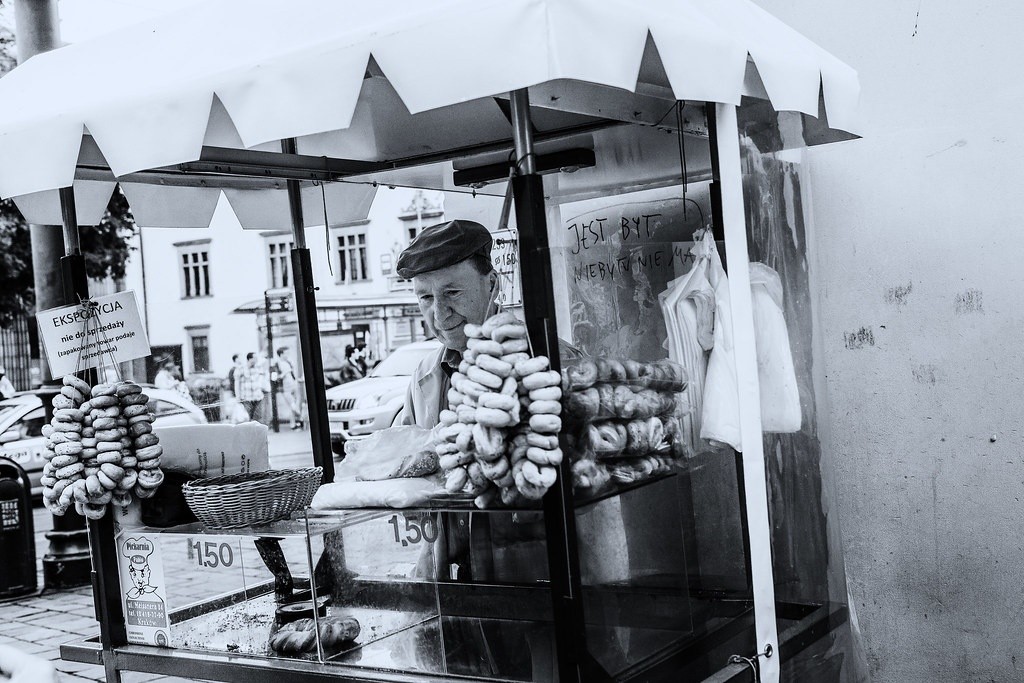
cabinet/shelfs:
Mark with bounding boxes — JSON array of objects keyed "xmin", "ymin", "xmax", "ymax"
[{"xmin": 113, "ymin": 448, "xmax": 847, "ymax": 683}]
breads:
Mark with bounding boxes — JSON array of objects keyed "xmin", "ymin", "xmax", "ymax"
[{"xmin": 268, "ymin": 613, "xmax": 360, "ymax": 655}]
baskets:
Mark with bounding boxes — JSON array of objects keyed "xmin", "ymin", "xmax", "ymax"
[{"xmin": 182, "ymin": 465, "xmax": 324, "ymax": 528}]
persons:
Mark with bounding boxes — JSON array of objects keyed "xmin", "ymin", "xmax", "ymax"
[
  {"xmin": 228, "ymin": 354, "xmax": 244, "ymax": 399},
  {"xmin": 0, "ymin": 368, "xmax": 16, "ymax": 403},
  {"xmin": 341, "ymin": 341, "xmax": 372, "ymax": 384},
  {"xmin": 396, "ymin": 218, "xmax": 635, "ymax": 588},
  {"xmin": 152, "ymin": 363, "xmax": 193, "ymax": 402},
  {"xmin": 269, "ymin": 345, "xmax": 308, "ymax": 433},
  {"xmin": 234, "ymin": 351, "xmax": 266, "ymax": 423}
]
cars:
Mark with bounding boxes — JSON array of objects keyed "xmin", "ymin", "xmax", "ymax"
[{"xmin": 0, "ymin": 386, "xmax": 210, "ymax": 488}]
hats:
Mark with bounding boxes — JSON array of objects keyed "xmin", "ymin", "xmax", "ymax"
[{"xmin": 395, "ymin": 220, "xmax": 493, "ymax": 279}]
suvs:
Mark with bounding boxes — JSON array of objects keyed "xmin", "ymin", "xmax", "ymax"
[{"xmin": 326, "ymin": 339, "xmax": 444, "ymax": 455}]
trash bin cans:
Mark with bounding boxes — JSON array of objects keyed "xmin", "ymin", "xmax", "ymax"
[{"xmin": 0, "ymin": 456, "xmax": 38, "ymax": 603}]
